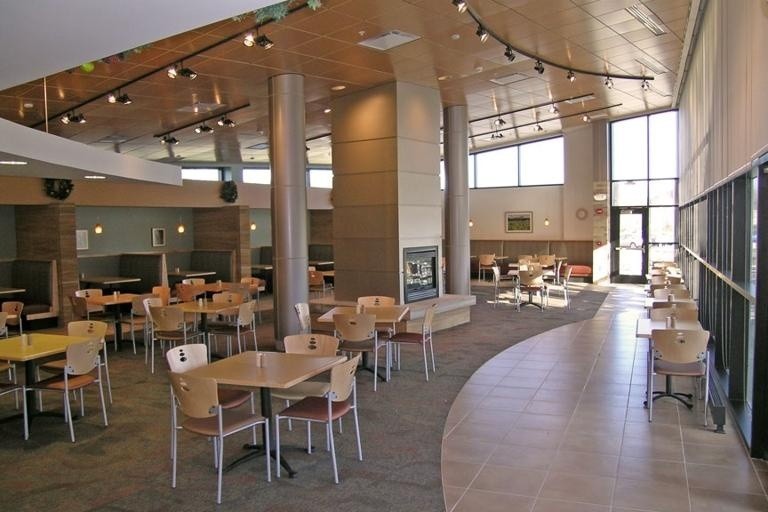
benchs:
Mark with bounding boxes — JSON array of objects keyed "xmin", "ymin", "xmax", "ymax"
[
  {"xmin": 9, "ymin": 257, "xmax": 59, "ymax": 321},
  {"xmin": 259, "ymin": 246, "xmax": 274, "ymax": 265},
  {"xmin": 119, "ymin": 254, "xmax": 167, "ymax": 296},
  {"xmin": 191, "ymin": 251, "xmax": 236, "ymax": 281},
  {"xmin": 309, "ymin": 245, "xmax": 334, "ymax": 260},
  {"xmin": 559, "ymin": 263, "xmax": 593, "ymax": 280}
]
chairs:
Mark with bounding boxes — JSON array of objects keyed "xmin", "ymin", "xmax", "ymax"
[
  {"xmin": 166, "ymin": 343, "xmax": 257, "ymax": 472},
  {"xmin": 271, "ymin": 334, "xmax": 342, "ymax": 451},
  {"xmin": 68, "ymin": 277, "xmax": 266, "ymax": 374},
  {"xmin": 309, "ymin": 267, "xmax": 334, "ymax": 297},
  {"xmin": 1, "ymin": 301, "xmax": 112, "ymax": 443},
  {"xmin": 470, "ymin": 254, "xmax": 572, "ymax": 313},
  {"xmin": 293, "ymin": 296, "xmax": 437, "ymax": 392},
  {"xmin": 634, "ymin": 260, "xmax": 713, "ymax": 430},
  {"xmin": 167, "ymin": 370, "xmax": 271, "ymax": 504},
  {"xmin": 275, "ymin": 352, "xmax": 362, "ymax": 485}
]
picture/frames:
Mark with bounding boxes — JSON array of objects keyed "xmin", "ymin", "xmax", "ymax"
[
  {"xmin": 503, "ymin": 210, "xmax": 533, "ymax": 234},
  {"xmin": 75, "ymin": 229, "xmax": 89, "ymax": 251},
  {"xmin": 151, "ymin": 227, "xmax": 166, "ymax": 248},
  {"xmin": 403, "ymin": 247, "xmax": 438, "ymax": 303}
]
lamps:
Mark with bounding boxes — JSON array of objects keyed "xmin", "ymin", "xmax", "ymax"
[
  {"xmin": 176, "ymin": 207, "xmax": 185, "ymax": 234},
  {"xmin": 496, "ymin": 104, "xmax": 559, "ymax": 126},
  {"xmin": 467, "ymin": 219, "xmax": 473, "ymax": 227},
  {"xmin": 543, "ymin": 217, "xmax": 549, "ymax": 226},
  {"xmin": 94, "ymin": 206, "xmax": 103, "ymax": 235},
  {"xmin": 250, "ymin": 221, "xmax": 256, "ymax": 231},
  {"xmin": 451, "ymin": 1, "xmax": 653, "ymax": 92},
  {"xmin": 162, "ymin": 115, "xmax": 238, "ymax": 146},
  {"xmin": 60, "ymin": 24, "xmax": 275, "ymax": 123},
  {"xmin": 490, "ymin": 114, "xmax": 590, "ymax": 140}
]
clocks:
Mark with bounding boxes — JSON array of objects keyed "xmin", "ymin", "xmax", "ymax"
[{"xmin": 576, "ymin": 208, "xmax": 589, "ymax": 221}]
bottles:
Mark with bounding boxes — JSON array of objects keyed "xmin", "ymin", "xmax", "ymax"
[
  {"xmin": 665, "ymin": 303, "xmax": 678, "ymax": 329},
  {"xmin": 198, "ymin": 298, "xmax": 207, "ymax": 307},
  {"xmin": 355, "ymin": 302, "xmax": 366, "ymax": 315},
  {"xmin": 255, "ymin": 351, "xmax": 264, "ymax": 367},
  {"xmin": 113, "ymin": 291, "xmax": 120, "ymax": 300},
  {"xmin": 21, "ymin": 334, "xmax": 34, "ymax": 347},
  {"xmin": 216, "ymin": 280, "xmax": 222, "ymax": 286}
]
